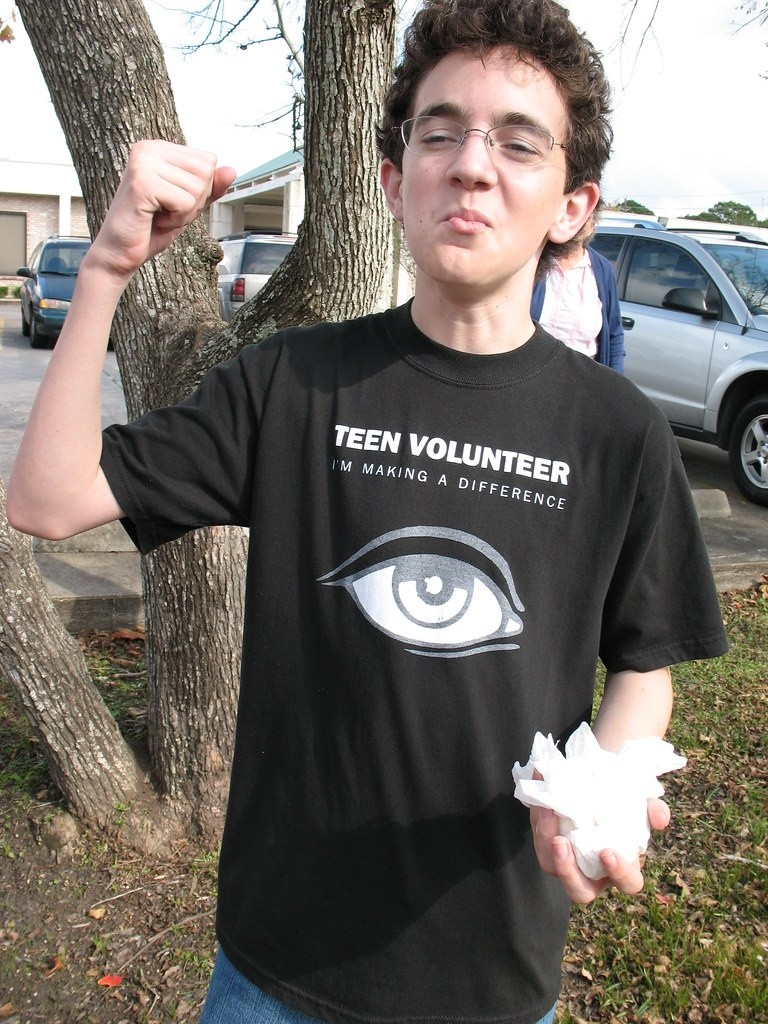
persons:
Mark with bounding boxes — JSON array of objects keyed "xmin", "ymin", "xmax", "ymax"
[
  {"xmin": 5, "ymin": 0, "xmax": 730, "ymax": 1024},
  {"xmin": 530, "ymin": 196, "xmax": 624, "ymax": 371}
]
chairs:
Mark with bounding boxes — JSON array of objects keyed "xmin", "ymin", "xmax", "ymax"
[
  {"xmin": 672, "ymin": 254, "xmax": 698, "ymax": 286},
  {"xmin": 624, "ymin": 244, "xmax": 673, "ymax": 307},
  {"xmin": 45, "ymin": 258, "xmax": 66, "ymax": 272}
]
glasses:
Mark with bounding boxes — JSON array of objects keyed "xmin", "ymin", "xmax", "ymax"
[{"xmin": 391, "ymin": 116, "xmax": 589, "ymax": 181}]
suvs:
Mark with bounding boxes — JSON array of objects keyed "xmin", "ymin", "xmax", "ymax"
[
  {"xmin": 16, "ymin": 235, "xmax": 117, "ymax": 349},
  {"xmin": 217, "ymin": 230, "xmax": 297, "ymax": 325},
  {"xmin": 581, "ymin": 223, "xmax": 768, "ymax": 510}
]
